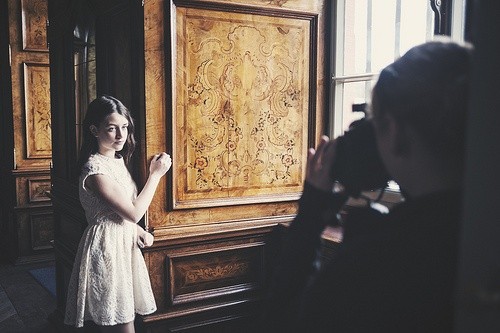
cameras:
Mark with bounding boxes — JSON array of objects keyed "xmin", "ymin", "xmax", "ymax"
[{"xmin": 327, "ymin": 99, "xmax": 392, "ymax": 200}]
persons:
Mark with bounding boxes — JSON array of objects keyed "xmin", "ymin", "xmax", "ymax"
[
  {"xmin": 258, "ymin": 35, "xmax": 500, "ymax": 333},
  {"xmin": 64, "ymin": 95, "xmax": 172, "ymax": 333}
]
